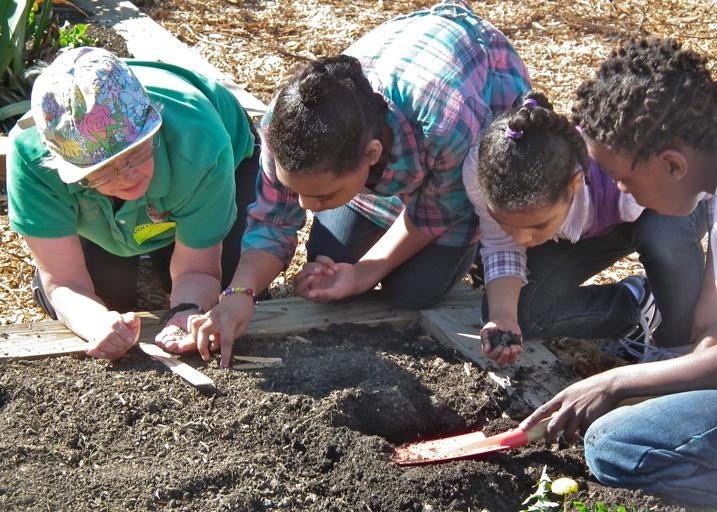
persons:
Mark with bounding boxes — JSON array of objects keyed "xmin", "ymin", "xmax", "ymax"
[
  {"xmin": 463, "ymin": 89, "xmax": 717, "ymax": 365},
  {"xmin": 517, "ymin": 40, "xmax": 716, "ymax": 512},
  {"xmin": 6, "ymin": 47, "xmax": 272, "ymax": 363},
  {"xmin": 185, "ymin": 0, "xmax": 534, "ymax": 371}
]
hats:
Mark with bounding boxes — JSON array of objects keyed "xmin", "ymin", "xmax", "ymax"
[{"xmin": 31, "ymin": 47, "xmax": 163, "ymax": 186}]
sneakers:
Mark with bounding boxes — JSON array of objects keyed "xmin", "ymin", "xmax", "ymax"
[{"xmin": 605, "ymin": 276, "xmax": 662, "ymax": 363}]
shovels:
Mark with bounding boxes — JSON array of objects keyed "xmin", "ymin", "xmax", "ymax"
[{"xmin": 389, "ymin": 414, "xmax": 561, "ymax": 465}]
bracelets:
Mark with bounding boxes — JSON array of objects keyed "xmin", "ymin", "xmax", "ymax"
[
  {"xmin": 218, "ymin": 284, "xmax": 257, "ymax": 306},
  {"xmin": 160, "ymin": 304, "xmax": 206, "ymax": 324}
]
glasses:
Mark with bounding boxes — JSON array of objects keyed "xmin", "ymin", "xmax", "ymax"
[{"xmin": 78, "ymin": 136, "xmax": 161, "ymax": 189}]
